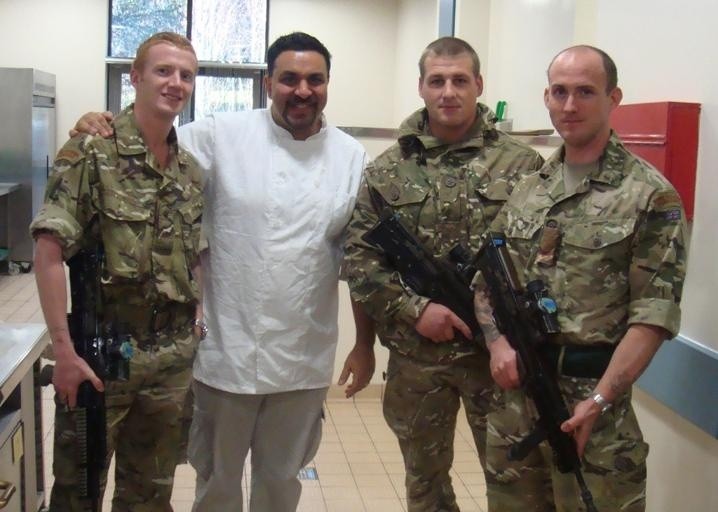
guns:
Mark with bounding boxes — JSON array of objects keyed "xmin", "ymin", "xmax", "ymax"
[
  {"xmin": 361, "ymin": 214, "xmax": 491, "ymax": 362},
  {"xmin": 473, "ymin": 232, "xmax": 596, "ymax": 510},
  {"xmin": 40, "ymin": 240, "xmax": 131, "ymax": 512}
]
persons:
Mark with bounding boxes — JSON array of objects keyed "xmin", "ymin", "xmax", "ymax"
[
  {"xmin": 68, "ymin": 33, "xmax": 375, "ymax": 512},
  {"xmin": 470, "ymin": 44, "xmax": 688, "ymax": 512},
  {"xmin": 29, "ymin": 33, "xmax": 210, "ymax": 512},
  {"xmin": 338, "ymin": 37, "xmax": 546, "ymax": 512}
]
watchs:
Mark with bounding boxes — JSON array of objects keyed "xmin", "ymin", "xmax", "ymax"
[
  {"xmin": 589, "ymin": 393, "xmax": 613, "ymax": 416},
  {"xmin": 195, "ymin": 319, "xmax": 208, "ymax": 340}
]
